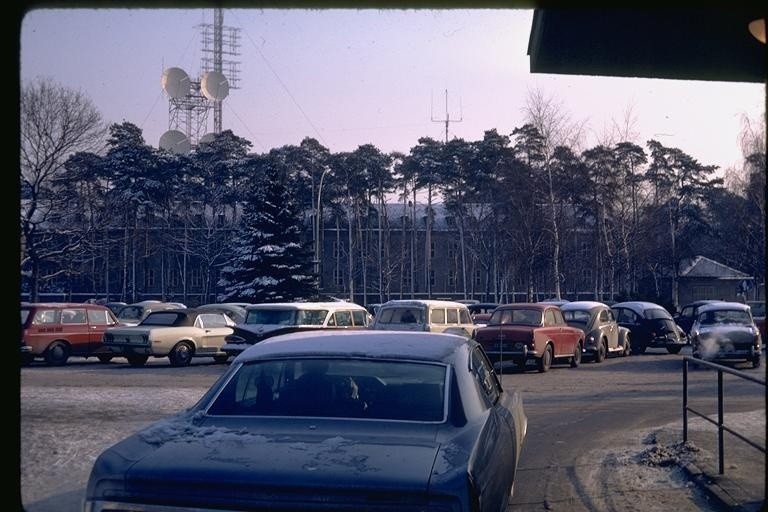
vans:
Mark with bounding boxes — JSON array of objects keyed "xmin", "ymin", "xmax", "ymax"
[
  {"xmin": 366, "ymin": 299, "xmax": 487, "ymax": 343},
  {"xmin": 220, "ymin": 300, "xmax": 375, "ymax": 359},
  {"xmin": 18, "ymin": 301, "xmax": 127, "ymax": 367}
]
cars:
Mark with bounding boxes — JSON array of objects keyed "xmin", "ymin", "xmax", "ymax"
[
  {"xmin": 556, "ymin": 300, "xmax": 631, "ymax": 363},
  {"xmin": 98, "ymin": 307, "xmax": 236, "ymax": 364},
  {"xmin": 688, "ymin": 302, "xmax": 763, "ymax": 368},
  {"xmin": 83, "ymin": 299, "xmax": 253, "ymax": 339},
  {"xmin": 474, "ymin": 303, "xmax": 586, "ymax": 372},
  {"xmin": 672, "ymin": 298, "xmax": 724, "ymax": 333},
  {"xmin": 82, "ymin": 329, "xmax": 529, "ymax": 512},
  {"xmin": 453, "ymin": 297, "xmax": 572, "ymax": 336},
  {"xmin": 610, "ymin": 301, "xmax": 687, "ymax": 355},
  {"xmin": 744, "ymin": 300, "xmax": 768, "ymax": 345}
]
